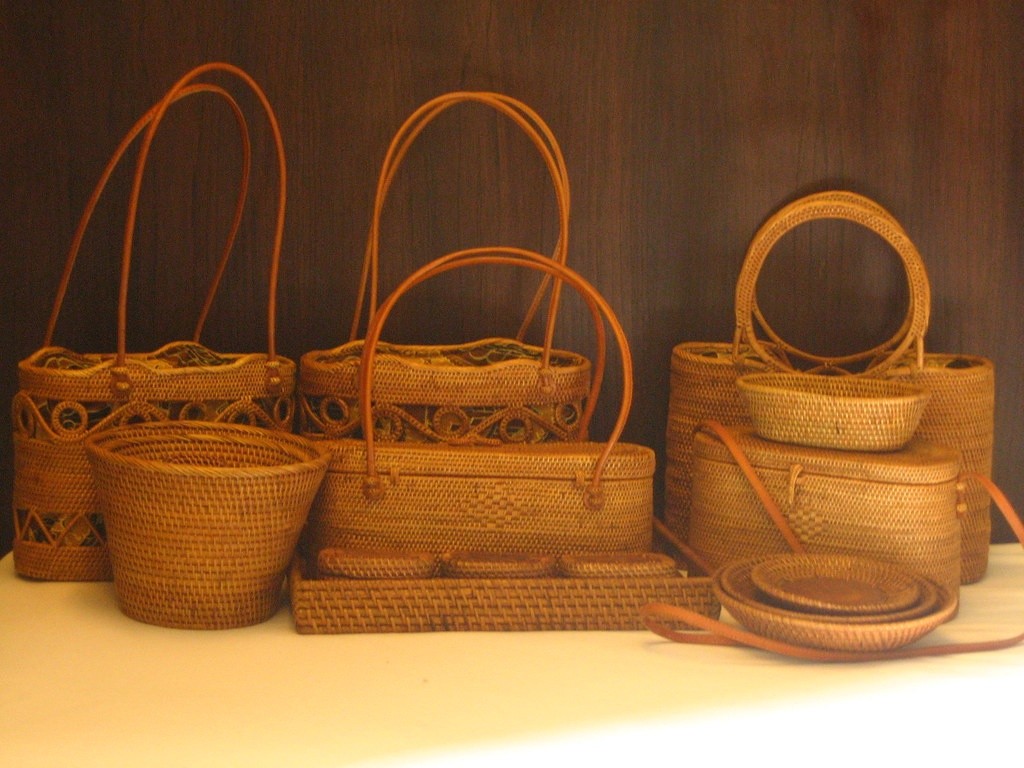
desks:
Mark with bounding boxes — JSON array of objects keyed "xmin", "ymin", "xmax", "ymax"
[{"xmin": 0, "ymin": 543, "xmax": 1024, "ymax": 768}]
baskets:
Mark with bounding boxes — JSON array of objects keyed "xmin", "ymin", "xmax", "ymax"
[
  {"xmin": 737, "ymin": 372, "xmax": 931, "ymax": 452},
  {"xmin": 688, "ymin": 426, "xmax": 962, "ymax": 598},
  {"xmin": 297, "ymin": 92, "xmax": 591, "ymax": 446},
  {"xmin": 752, "ymin": 557, "xmax": 923, "ymax": 615},
  {"xmin": 722, "ymin": 559, "xmax": 938, "ymax": 624},
  {"xmin": 11, "ymin": 61, "xmax": 297, "ymax": 582},
  {"xmin": 559, "ymin": 553, "xmax": 676, "ymax": 578},
  {"xmin": 306, "ymin": 247, "xmax": 655, "ymax": 578},
  {"xmin": 288, "ymin": 559, "xmax": 721, "ymax": 634},
  {"xmin": 443, "ymin": 552, "xmax": 556, "ymax": 578},
  {"xmin": 663, "ymin": 190, "xmax": 995, "ymax": 585},
  {"xmin": 319, "ymin": 549, "xmax": 434, "ymax": 578},
  {"xmin": 713, "ymin": 554, "xmax": 959, "ymax": 652},
  {"xmin": 85, "ymin": 420, "xmax": 331, "ymax": 630}
]
